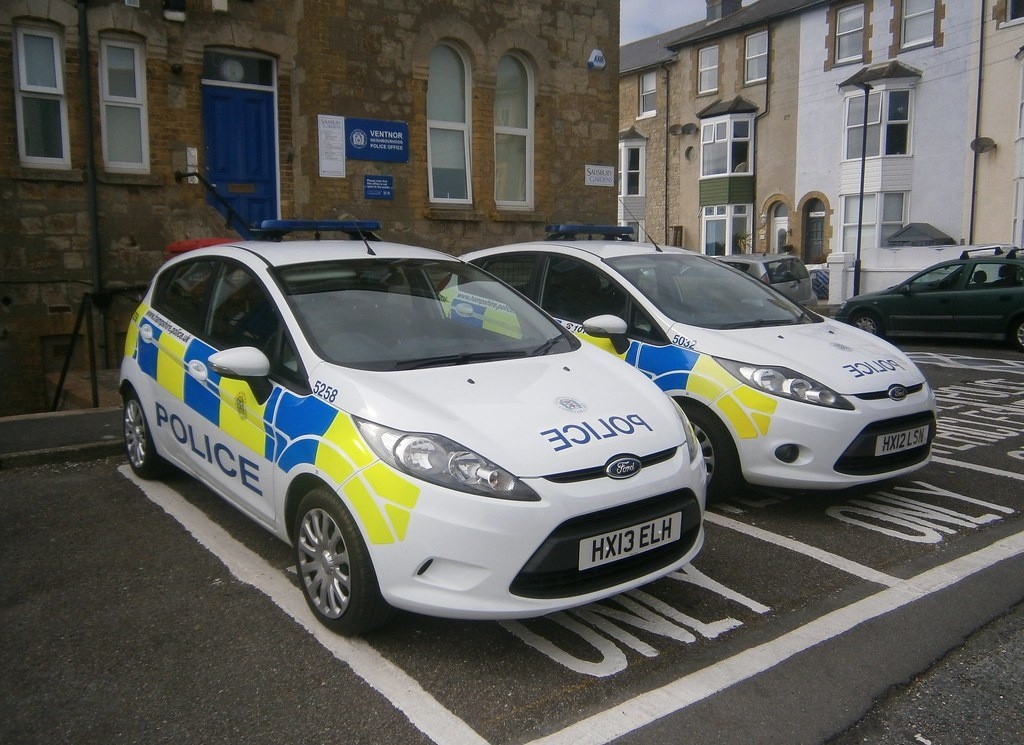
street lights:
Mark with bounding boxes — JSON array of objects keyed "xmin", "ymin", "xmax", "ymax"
[{"xmin": 854, "ymin": 82, "xmax": 873, "ymax": 296}]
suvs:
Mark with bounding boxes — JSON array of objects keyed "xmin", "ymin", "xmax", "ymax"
[{"xmin": 711, "ymin": 254, "xmax": 818, "ymax": 313}]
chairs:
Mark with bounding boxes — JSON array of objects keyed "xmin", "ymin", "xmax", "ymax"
[
  {"xmin": 992, "ymin": 266, "xmax": 1008, "ymax": 285},
  {"xmin": 550, "ymin": 270, "xmax": 615, "ymax": 318},
  {"xmin": 973, "ymin": 270, "xmax": 988, "ymax": 288}
]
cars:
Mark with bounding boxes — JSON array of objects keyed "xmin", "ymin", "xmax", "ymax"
[
  {"xmin": 836, "ymin": 258, "xmax": 1024, "ymax": 349},
  {"xmin": 438, "ymin": 237, "xmax": 935, "ymax": 502},
  {"xmin": 117, "ymin": 238, "xmax": 709, "ymax": 636}
]
